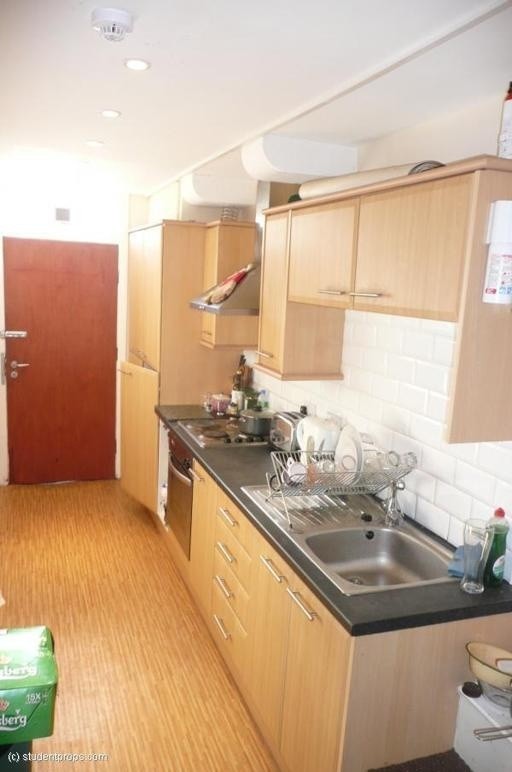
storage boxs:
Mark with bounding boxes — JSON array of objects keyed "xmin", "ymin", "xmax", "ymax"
[{"xmin": 0, "ymin": 625, "xmax": 57, "ymax": 744}]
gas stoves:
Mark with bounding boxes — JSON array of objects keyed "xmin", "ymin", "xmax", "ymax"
[{"xmin": 167, "ymin": 418, "xmax": 266, "ymax": 477}]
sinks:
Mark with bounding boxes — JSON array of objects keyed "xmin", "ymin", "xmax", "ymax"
[{"xmin": 305, "ymin": 525, "xmax": 463, "ymax": 597}]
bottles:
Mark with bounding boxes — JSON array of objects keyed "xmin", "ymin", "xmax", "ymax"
[{"xmin": 257, "ymin": 390, "xmax": 269, "ymax": 408}]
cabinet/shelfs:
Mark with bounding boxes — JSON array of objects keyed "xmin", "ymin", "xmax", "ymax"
[
  {"xmin": 451, "ymin": 683, "xmax": 511, "ymax": 772},
  {"xmin": 116, "ymin": 217, "xmax": 243, "ymax": 509},
  {"xmin": 252, "ymin": 207, "xmax": 346, "ymax": 380},
  {"xmin": 199, "ymin": 220, "xmax": 258, "ymax": 350},
  {"xmin": 183, "ymin": 457, "xmax": 217, "ymax": 620},
  {"xmin": 203, "ymin": 485, "xmax": 263, "ymax": 683},
  {"xmin": 287, "ymin": 156, "xmax": 512, "ymax": 321},
  {"xmin": 239, "ymin": 534, "xmax": 511, "ymax": 771}
]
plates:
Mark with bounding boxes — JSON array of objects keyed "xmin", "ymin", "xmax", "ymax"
[{"xmin": 335, "ymin": 422, "xmax": 366, "ymax": 487}]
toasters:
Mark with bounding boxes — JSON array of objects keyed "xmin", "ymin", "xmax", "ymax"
[{"xmin": 267, "ymin": 408, "xmax": 309, "ymax": 452}]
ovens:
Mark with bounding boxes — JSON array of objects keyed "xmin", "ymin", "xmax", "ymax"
[{"xmin": 164, "ymin": 447, "xmax": 194, "ymax": 562}]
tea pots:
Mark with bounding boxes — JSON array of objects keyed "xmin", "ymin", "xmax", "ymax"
[{"xmin": 293, "ymin": 416, "xmax": 340, "ymax": 464}]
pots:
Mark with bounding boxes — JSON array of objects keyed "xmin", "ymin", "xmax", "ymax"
[
  {"xmin": 228, "ymin": 407, "xmax": 275, "ymax": 435},
  {"xmin": 210, "ymin": 393, "xmax": 231, "ymax": 412}
]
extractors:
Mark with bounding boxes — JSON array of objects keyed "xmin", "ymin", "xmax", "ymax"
[{"xmin": 191, "ymin": 181, "xmax": 300, "ymax": 313}]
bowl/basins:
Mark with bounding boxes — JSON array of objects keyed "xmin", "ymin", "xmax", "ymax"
[{"xmin": 464, "ymin": 640, "xmax": 511, "ymax": 691}]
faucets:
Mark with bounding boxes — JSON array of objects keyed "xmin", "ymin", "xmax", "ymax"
[{"xmin": 382, "ymin": 478, "xmax": 409, "ymax": 528}]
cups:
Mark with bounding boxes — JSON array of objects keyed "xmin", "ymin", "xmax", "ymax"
[
  {"xmin": 313, "ymin": 459, "xmax": 333, "ymax": 484},
  {"xmin": 460, "ymin": 520, "xmax": 496, "ymax": 595},
  {"xmin": 281, "ymin": 458, "xmax": 308, "ymax": 488},
  {"xmin": 365, "ymin": 450, "xmax": 419, "ymax": 473}
]
